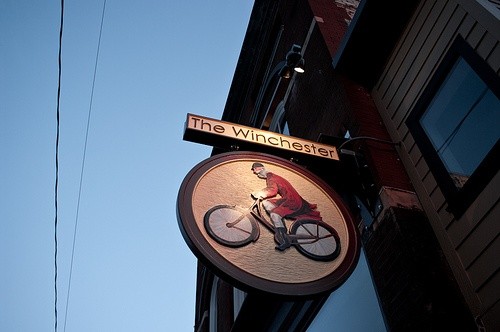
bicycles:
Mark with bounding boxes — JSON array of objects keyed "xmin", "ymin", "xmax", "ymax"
[{"xmin": 204, "ymin": 190, "xmax": 341, "ymax": 262}]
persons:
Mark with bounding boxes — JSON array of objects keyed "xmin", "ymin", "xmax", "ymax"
[{"xmin": 251, "ymin": 163, "xmax": 324, "ymax": 252}]
